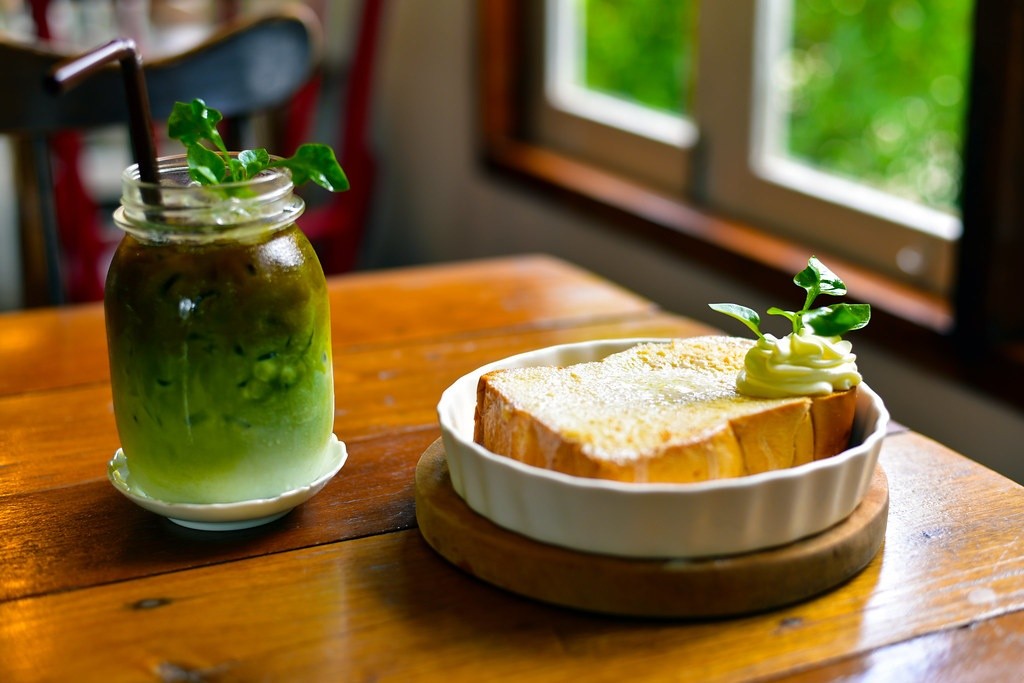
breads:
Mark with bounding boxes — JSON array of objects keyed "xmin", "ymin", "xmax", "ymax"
[{"xmin": 474, "ymin": 333, "xmax": 858, "ymax": 485}]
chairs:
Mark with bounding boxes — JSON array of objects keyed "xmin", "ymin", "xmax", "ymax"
[{"xmin": 0, "ymin": 4, "xmax": 323, "ymax": 308}]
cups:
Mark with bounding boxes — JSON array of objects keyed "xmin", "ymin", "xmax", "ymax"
[{"xmin": 104, "ymin": 151, "xmax": 335, "ymax": 505}]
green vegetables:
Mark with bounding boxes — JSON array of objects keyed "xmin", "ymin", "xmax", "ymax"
[
  {"xmin": 169, "ymin": 100, "xmax": 351, "ymax": 198},
  {"xmin": 711, "ymin": 256, "xmax": 871, "ymax": 344}
]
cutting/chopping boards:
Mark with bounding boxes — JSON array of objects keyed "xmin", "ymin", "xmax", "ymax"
[{"xmin": 414, "ymin": 435, "xmax": 888, "ymax": 618}]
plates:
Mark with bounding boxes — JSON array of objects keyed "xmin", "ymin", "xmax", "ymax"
[
  {"xmin": 436, "ymin": 338, "xmax": 891, "ymax": 558},
  {"xmin": 107, "ymin": 433, "xmax": 348, "ymax": 531}
]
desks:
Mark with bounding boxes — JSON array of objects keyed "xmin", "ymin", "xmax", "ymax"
[{"xmin": 0, "ymin": 251, "xmax": 1024, "ymax": 683}]
